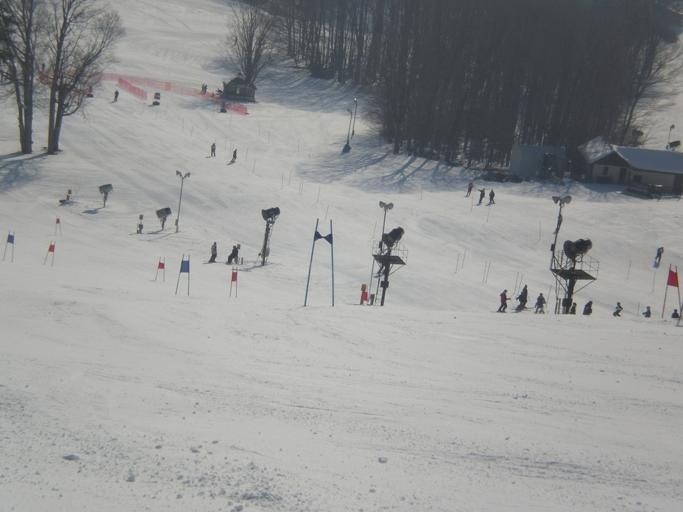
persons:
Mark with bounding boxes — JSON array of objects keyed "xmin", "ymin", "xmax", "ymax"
[
  {"xmin": 232, "ymin": 148, "xmax": 237, "ymax": 161},
  {"xmin": 582, "ymin": 300, "xmax": 593, "ymax": 315},
  {"xmin": 474, "ymin": 188, "xmax": 485, "ymax": 206},
  {"xmin": 534, "ymin": 292, "xmax": 546, "ymax": 313},
  {"xmin": 497, "ymin": 289, "xmax": 511, "ymax": 313},
  {"xmin": 570, "ymin": 302, "xmax": 577, "ymax": 314},
  {"xmin": 488, "ymin": 189, "xmax": 494, "ymax": 203},
  {"xmin": 612, "ymin": 301, "xmax": 623, "ymax": 316},
  {"xmin": 226, "ymin": 245, "xmax": 236, "ymax": 264},
  {"xmin": 464, "ymin": 183, "xmax": 472, "ymax": 197},
  {"xmin": 641, "ymin": 306, "xmax": 651, "ymax": 318},
  {"xmin": 210, "ymin": 143, "xmax": 215, "ymax": 157},
  {"xmin": 208, "ymin": 241, "xmax": 217, "ymax": 263},
  {"xmin": 113, "ymin": 90, "xmax": 118, "ymax": 101},
  {"xmin": 654, "ymin": 246, "xmax": 664, "ymax": 262},
  {"xmin": 515, "ymin": 292, "xmax": 523, "ymax": 308},
  {"xmin": 521, "ymin": 283, "xmax": 527, "ymax": 306},
  {"xmin": 671, "ymin": 309, "xmax": 680, "ymax": 319}
]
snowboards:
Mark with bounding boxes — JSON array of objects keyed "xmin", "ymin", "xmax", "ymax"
[{"xmin": 226, "ymin": 262, "xmax": 233, "ymax": 265}]
